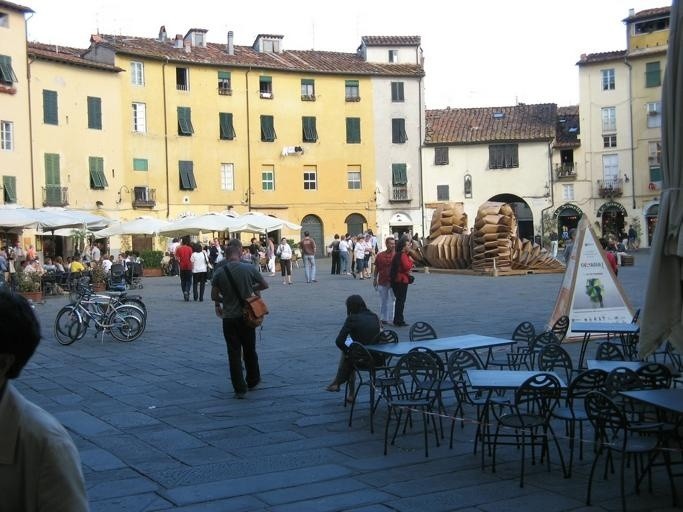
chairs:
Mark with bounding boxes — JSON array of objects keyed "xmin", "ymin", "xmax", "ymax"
[
  {"xmin": 259, "ymin": 256, "xmax": 298, "ymax": 273},
  {"xmin": 42, "ymin": 261, "xmax": 144, "ymax": 295}
]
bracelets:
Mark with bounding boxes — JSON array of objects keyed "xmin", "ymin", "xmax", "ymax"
[{"xmin": 373, "ymin": 277, "xmax": 377, "ymax": 280}]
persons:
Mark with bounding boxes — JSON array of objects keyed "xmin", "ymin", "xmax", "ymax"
[
  {"xmin": 0, "ymin": 235, "xmax": 143, "ymax": 297},
  {"xmin": 203, "ymin": 237, "xmax": 226, "ymax": 288},
  {"xmin": 160, "ymin": 237, "xmax": 180, "ymax": 277},
  {"xmin": 275, "ymin": 238, "xmax": 293, "ymax": 284},
  {"xmin": 190, "ymin": 243, "xmax": 213, "ymax": 302},
  {"xmin": 548, "ymin": 225, "xmax": 577, "ymax": 270},
  {"xmin": 390, "ymin": 237, "xmax": 414, "ymax": 326},
  {"xmin": 373, "ymin": 237, "xmax": 397, "ymax": 326},
  {"xmin": 329, "ymin": 229, "xmax": 379, "ymax": 281},
  {"xmin": 0, "ymin": 284, "xmax": 89, "ymax": 511},
  {"xmin": 249, "ymin": 237, "xmax": 262, "ymax": 270},
  {"xmin": 299, "ymin": 231, "xmax": 318, "ymax": 283},
  {"xmin": 175, "ymin": 235, "xmax": 193, "ymax": 302},
  {"xmin": 211, "ymin": 245, "xmax": 268, "ymax": 400},
  {"xmin": 325, "ymin": 295, "xmax": 383, "ymax": 403},
  {"xmin": 265, "ymin": 237, "xmax": 276, "ymax": 277},
  {"xmin": 598, "ymin": 223, "xmax": 639, "ymax": 277},
  {"xmin": 213, "ymin": 239, "xmax": 262, "ymax": 317}
]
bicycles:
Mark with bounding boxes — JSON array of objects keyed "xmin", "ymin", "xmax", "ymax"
[{"xmin": 52, "ymin": 275, "xmax": 149, "ymax": 345}]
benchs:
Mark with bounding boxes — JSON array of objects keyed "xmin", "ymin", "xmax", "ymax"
[{"xmin": 615, "ymin": 256, "xmax": 633, "ymax": 266}]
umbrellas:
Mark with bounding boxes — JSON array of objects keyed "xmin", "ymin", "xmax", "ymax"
[
  {"xmin": 0, "ymin": 206, "xmax": 108, "ymax": 241},
  {"xmin": 93, "ymin": 211, "xmax": 304, "ymax": 243}
]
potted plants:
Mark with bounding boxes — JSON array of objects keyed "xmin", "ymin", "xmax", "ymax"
[
  {"xmin": 89, "ymin": 262, "xmax": 109, "ymax": 293},
  {"xmin": 12, "ymin": 271, "xmax": 43, "ymax": 302}
]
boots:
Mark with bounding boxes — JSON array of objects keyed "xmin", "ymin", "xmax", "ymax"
[
  {"xmin": 286, "ymin": 275, "xmax": 293, "ymax": 284},
  {"xmin": 281, "ymin": 276, "xmax": 286, "ymax": 285}
]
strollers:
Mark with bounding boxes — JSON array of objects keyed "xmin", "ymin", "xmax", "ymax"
[
  {"xmin": 108, "ymin": 264, "xmax": 125, "ymax": 283},
  {"xmin": 124, "ymin": 262, "xmax": 143, "ymax": 289}
]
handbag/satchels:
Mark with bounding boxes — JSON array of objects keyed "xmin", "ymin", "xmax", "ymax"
[
  {"xmin": 407, "ymin": 275, "xmax": 415, "ymax": 284},
  {"xmin": 241, "ymin": 294, "xmax": 270, "ymax": 327},
  {"xmin": 206, "ymin": 264, "xmax": 213, "ymax": 273}
]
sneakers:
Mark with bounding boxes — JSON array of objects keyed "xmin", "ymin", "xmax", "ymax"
[
  {"xmin": 230, "ymin": 392, "xmax": 248, "ymax": 400},
  {"xmin": 246, "ymin": 377, "xmax": 261, "ymax": 391}
]
